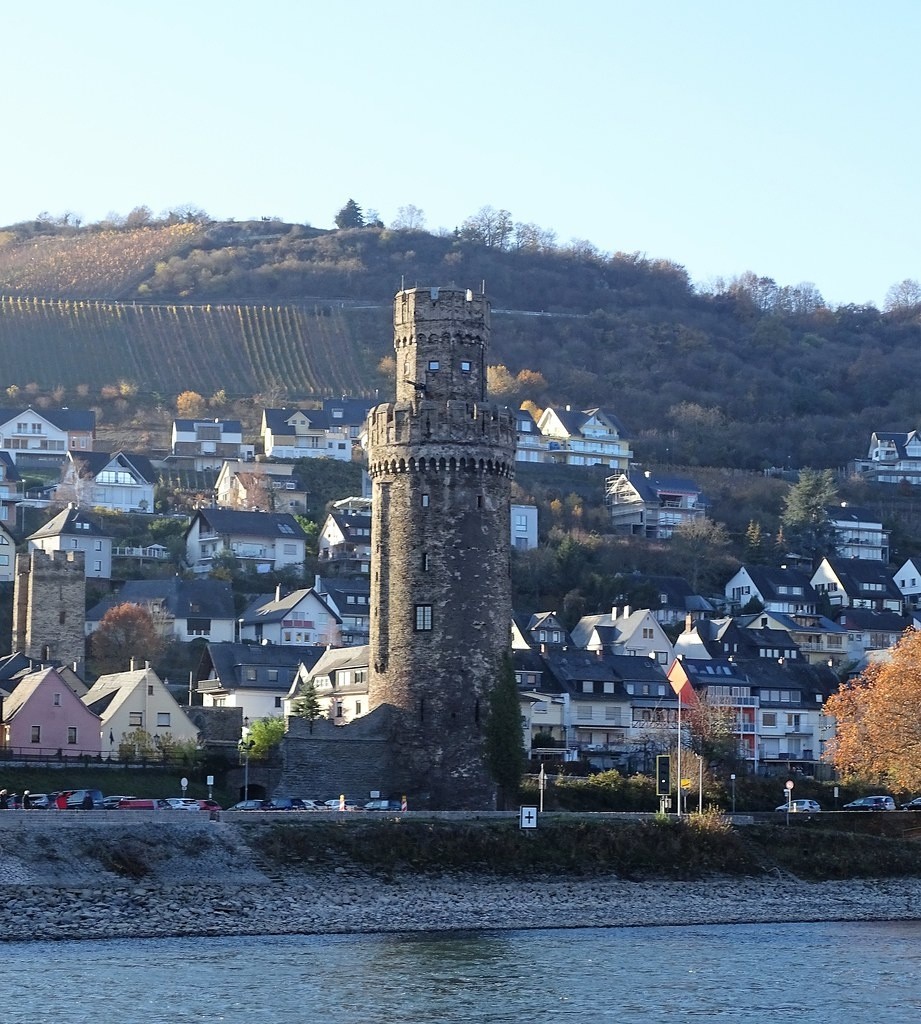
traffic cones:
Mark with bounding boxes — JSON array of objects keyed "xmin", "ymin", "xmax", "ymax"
[
  {"xmin": 401, "ymin": 796, "xmax": 408, "ymax": 812},
  {"xmin": 337, "ymin": 793, "xmax": 347, "ymax": 811}
]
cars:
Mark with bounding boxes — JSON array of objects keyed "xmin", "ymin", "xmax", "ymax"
[
  {"xmin": 844, "ymin": 794, "xmax": 896, "ymax": 811},
  {"xmin": 3, "ymin": 785, "xmax": 401, "ymax": 813},
  {"xmin": 901, "ymin": 796, "xmax": 921, "ymax": 810},
  {"xmin": 775, "ymin": 799, "xmax": 821, "ymax": 812}
]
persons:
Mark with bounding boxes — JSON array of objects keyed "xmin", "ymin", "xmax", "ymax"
[
  {"xmin": 82, "ymin": 792, "xmax": 94, "ymax": 810},
  {"xmin": 21, "ymin": 789, "xmax": 32, "ymax": 809},
  {"xmin": 0, "ymin": 789, "xmax": 10, "ymax": 808},
  {"xmin": 55, "ymin": 790, "xmax": 70, "ymax": 809}
]
